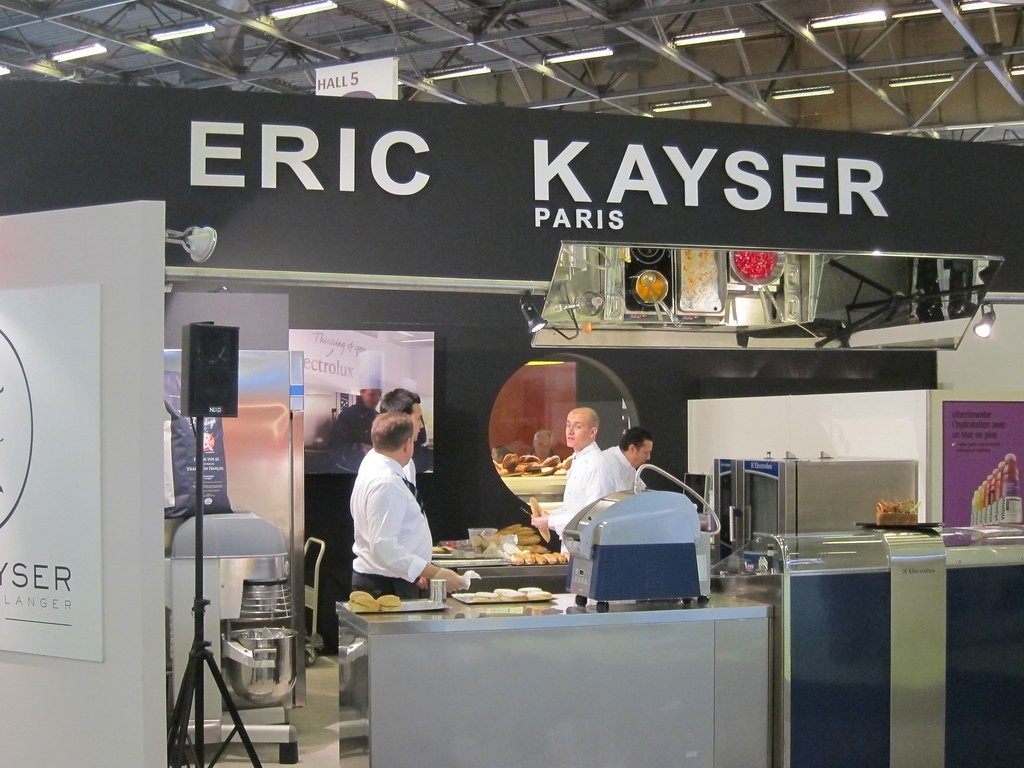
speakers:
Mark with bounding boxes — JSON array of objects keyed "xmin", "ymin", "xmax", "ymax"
[{"xmin": 180, "ymin": 323, "xmax": 239, "ymax": 418}]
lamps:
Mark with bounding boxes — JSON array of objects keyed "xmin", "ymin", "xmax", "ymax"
[
  {"xmin": 0, "ymin": 0, "xmax": 1024, "ymax": 114},
  {"xmin": 973, "ymin": 300, "xmax": 997, "ymax": 337},
  {"xmin": 164, "ymin": 226, "xmax": 218, "ymax": 262},
  {"xmin": 519, "ymin": 289, "xmax": 549, "ymax": 333}
]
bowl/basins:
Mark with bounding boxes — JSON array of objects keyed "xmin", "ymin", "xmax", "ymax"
[
  {"xmin": 635, "ymin": 269, "xmax": 669, "ymax": 303},
  {"xmin": 729, "ymin": 250, "xmax": 787, "ymax": 288},
  {"xmin": 226, "ymin": 627, "xmax": 299, "ymax": 708}
]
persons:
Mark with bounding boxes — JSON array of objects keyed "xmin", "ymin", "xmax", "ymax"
[
  {"xmin": 348, "ymin": 389, "xmax": 466, "ymax": 603},
  {"xmin": 491, "ymin": 407, "xmax": 653, "ymax": 554},
  {"xmin": 329, "ymin": 352, "xmax": 385, "ymax": 468}
]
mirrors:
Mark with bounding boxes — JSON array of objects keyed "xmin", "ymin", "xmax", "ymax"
[{"xmin": 530, "ymin": 240, "xmax": 1005, "ymax": 352}]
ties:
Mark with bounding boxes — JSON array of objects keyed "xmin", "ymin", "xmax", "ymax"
[{"xmin": 403, "ymin": 477, "xmax": 424, "ymax": 515}]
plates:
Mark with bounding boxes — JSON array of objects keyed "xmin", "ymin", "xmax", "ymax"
[{"xmin": 854, "ymin": 521, "xmax": 943, "ymax": 528}]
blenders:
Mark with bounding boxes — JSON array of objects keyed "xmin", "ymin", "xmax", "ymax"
[{"xmin": 164, "ymin": 511, "xmax": 299, "ymax": 765}]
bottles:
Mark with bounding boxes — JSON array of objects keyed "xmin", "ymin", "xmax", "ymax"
[{"xmin": 970, "ymin": 453, "xmax": 1023, "ymax": 526}]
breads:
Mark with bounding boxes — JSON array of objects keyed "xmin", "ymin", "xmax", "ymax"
[
  {"xmin": 493, "ymin": 454, "xmax": 574, "ymax": 477},
  {"xmin": 529, "ymin": 497, "xmax": 550, "ymax": 542},
  {"xmin": 348, "ymin": 591, "xmax": 403, "ymax": 611},
  {"xmin": 432, "ymin": 524, "xmax": 567, "ymax": 602}
]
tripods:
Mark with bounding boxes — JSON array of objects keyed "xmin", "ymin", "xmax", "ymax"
[{"xmin": 166, "ymin": 416, "xmax": 263, "ymax": 768}]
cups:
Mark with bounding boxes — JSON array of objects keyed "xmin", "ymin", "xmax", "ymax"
[
  {"xmin": 428, "ymin": 579, "xmax": 447, "ymax": 602},
  {"xmin": 467, "ymin": 527, "xmax": 498, "ymax": 555}
]
